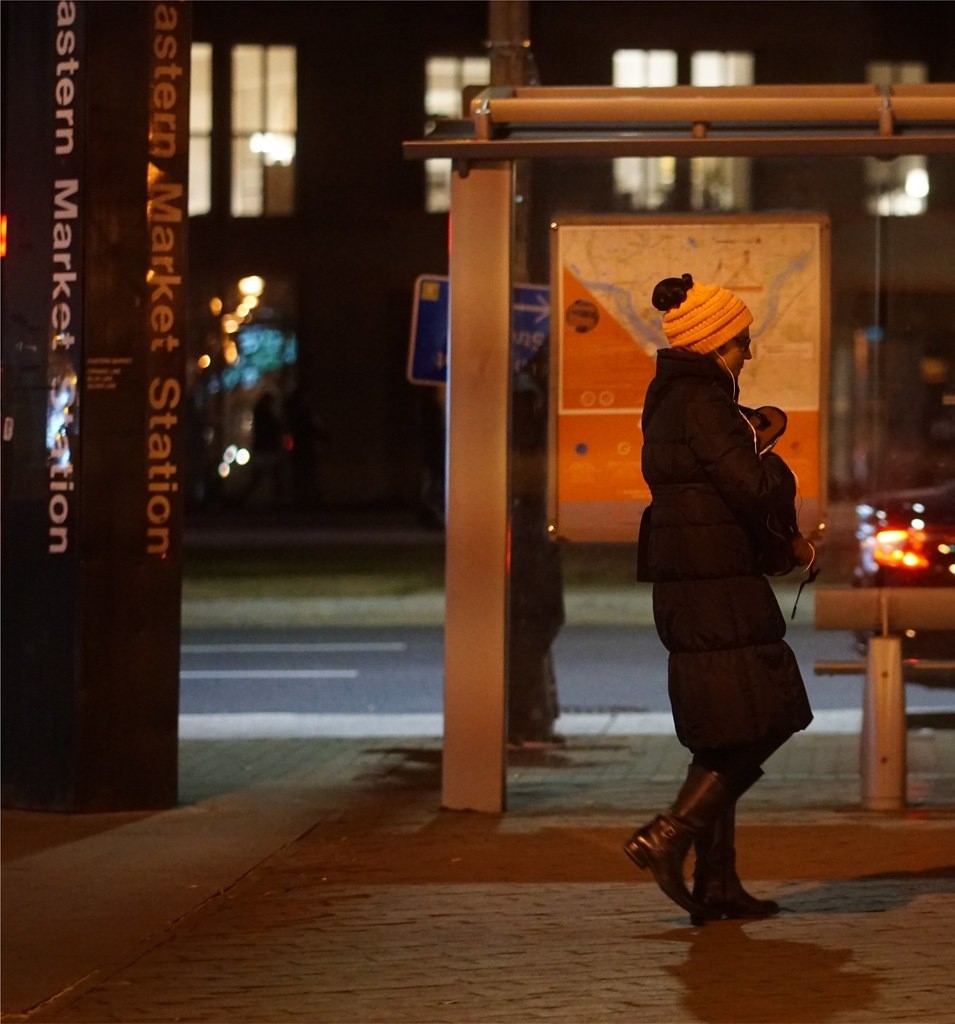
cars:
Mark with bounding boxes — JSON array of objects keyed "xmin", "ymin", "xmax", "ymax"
[{"xmin": 851, "ymin": 477, "xmax": 955, "ymax": 690}]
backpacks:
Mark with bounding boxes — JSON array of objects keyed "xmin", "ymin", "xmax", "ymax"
[{"xmin": 733, "ymin": 402, "xmax": 824, "ymax": 618}]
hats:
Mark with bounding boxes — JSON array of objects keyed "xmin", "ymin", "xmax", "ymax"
[{"xmin": 651, "ymin": 273, "xmax": 754, "ymax": 357}]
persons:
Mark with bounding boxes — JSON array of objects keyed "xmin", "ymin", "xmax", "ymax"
[{"xmin": 621, "ymin": 272, "xmax": 822, "ymax": 926}]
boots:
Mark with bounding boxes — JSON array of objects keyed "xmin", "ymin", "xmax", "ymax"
[
  {"xmin": 620, "ymin": 765, "xmax": 741, "ymax": 921},
  {"xmin": 692, "ymin": 805, "xmax": 781, "ymax": 927}
]
